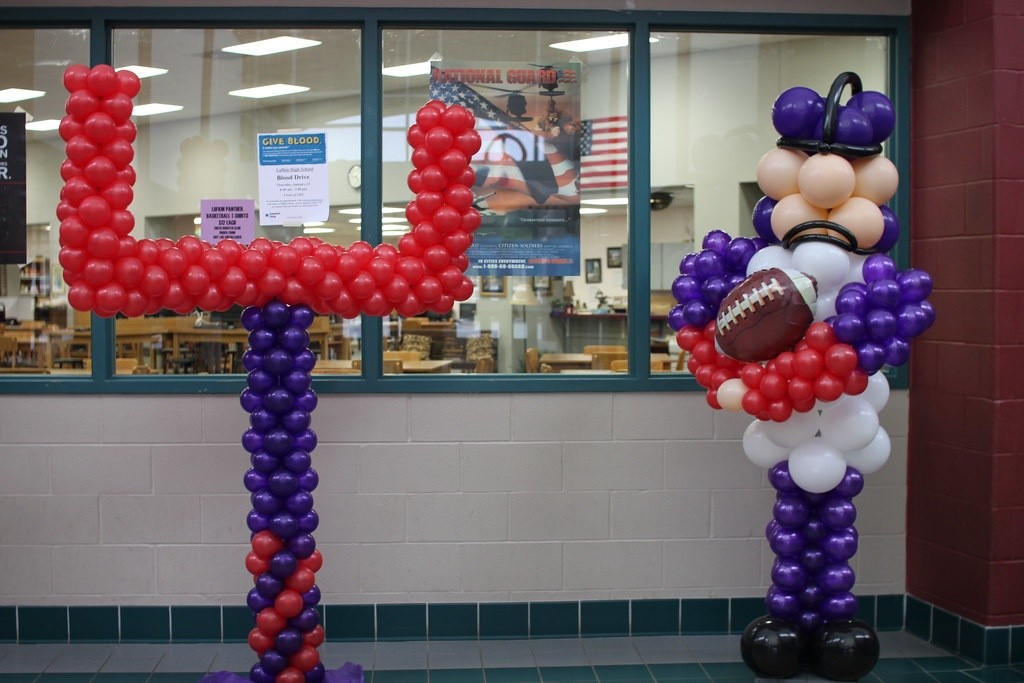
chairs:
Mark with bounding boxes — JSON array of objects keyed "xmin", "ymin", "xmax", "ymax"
[{"xmin": 1, "ymin": 311, "xmax": 688, "ymax": 376}]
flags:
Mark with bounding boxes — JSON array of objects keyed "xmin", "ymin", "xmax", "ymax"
[
  {"xmin": 427, "ymin": 67, "xmax": 582, "ymax": 204},
  {"xmin": 579, "ymin": 115, "xmax": 629, "ymax": 189}
]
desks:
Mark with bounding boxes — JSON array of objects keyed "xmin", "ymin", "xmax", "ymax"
[
  {"xmin": 551, "ymin": 310, "xmax": 670, "ymax": 353},
  {"xmin": 1, "ymin": 293, "xmax": 75, "ymax": 356}
]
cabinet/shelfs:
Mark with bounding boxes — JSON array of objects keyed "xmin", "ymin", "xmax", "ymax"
[{"xmin": 621, "ymin": 241, "xmax": 696, "ymax": 291}]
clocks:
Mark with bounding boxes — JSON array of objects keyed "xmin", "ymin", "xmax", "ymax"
[{"xmin": 347, "ymin": 164, "xmax": 362, "ymax": 190}]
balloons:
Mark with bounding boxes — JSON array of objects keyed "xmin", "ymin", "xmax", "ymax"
[
  {"xmin": 663, "ymin": 71, "xmax": 938, "ymax": 683},
  {"xmin": 54, "ymin": 63, "xmax": 483, "ymax": 682}
]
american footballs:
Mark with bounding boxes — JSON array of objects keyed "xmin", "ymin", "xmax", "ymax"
[{"xmin": 714, "ymin": 266, "xmax": 819, "ymax": 364}]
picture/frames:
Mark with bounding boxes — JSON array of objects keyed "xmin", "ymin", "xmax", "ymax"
[
  {"xmin": 607, "ymin": 247, "xmax": 623, "ymax": 268},
  {"xmin": 585, "ymin": 259, "xmax": 602, "ymax": 283},
  {"xmin": 49, "ymin": 262, "xmax": 67, "ymax": 299},
  {"xmin": 531, "ymin": 276, "xmax": 553, "ymax": 291},
  {"xmin": 481, "ymin": 276, "xmax": 507, "ymax": 297}
]
